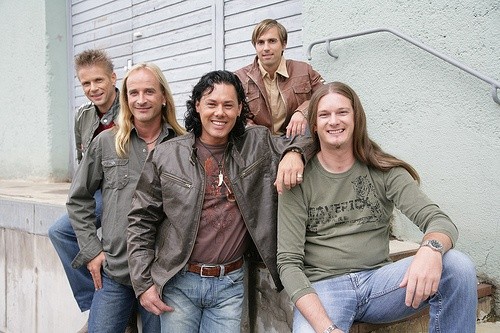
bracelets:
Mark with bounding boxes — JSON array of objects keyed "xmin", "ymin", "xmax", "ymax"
[
  {"xmin": 322, "ymin": 324, "xmax": 338, "ymax": 333},
  {"xmin": 287, "ymin": 147, "xmax": 302, "ymax": 153}
]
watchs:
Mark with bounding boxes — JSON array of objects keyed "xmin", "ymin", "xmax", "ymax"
[{"xmin": 420, "ymin": 239, "xmax": 444, "ymax": 257}]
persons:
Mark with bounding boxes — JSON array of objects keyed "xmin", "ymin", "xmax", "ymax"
[
  {"xmin": 276, "ymin": 80, "xmax": 478, "ymax": 333},
  {"xmin": 48, "ymin": 51, "xmax": 122, "ymax": 333},
  {"xmin": 65, "ymin": 61, "xmax": 190, "ymax": 333},
  {"xmin": 233, "ymin": 18, "xmax": 328, "ymax": 140},
  {"xmin": 125, "ymin": 70, "xmax": 320, "ymax": 333}
]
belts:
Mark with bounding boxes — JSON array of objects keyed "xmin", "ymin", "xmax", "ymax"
[{"xmin": 188, "ymin": 256, "xmax": 244, "ymax": 278}]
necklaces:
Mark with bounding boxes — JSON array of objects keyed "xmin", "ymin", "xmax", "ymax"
[
  {"xmin": 197, "ymin": 134, "xmax": 237, "ymax": 203},
  {"xmin": 146, "ymin": 123, "xmax": 163, "ymax": 145}
]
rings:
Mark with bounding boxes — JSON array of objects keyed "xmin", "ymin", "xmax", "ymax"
[
  {"xmin": 90, "ymin": 272, "xmax": 92, "ymax": 274},
  {"xmin": 296, "ymin": 174, "xmax": 303, "ymax": 178}
]
